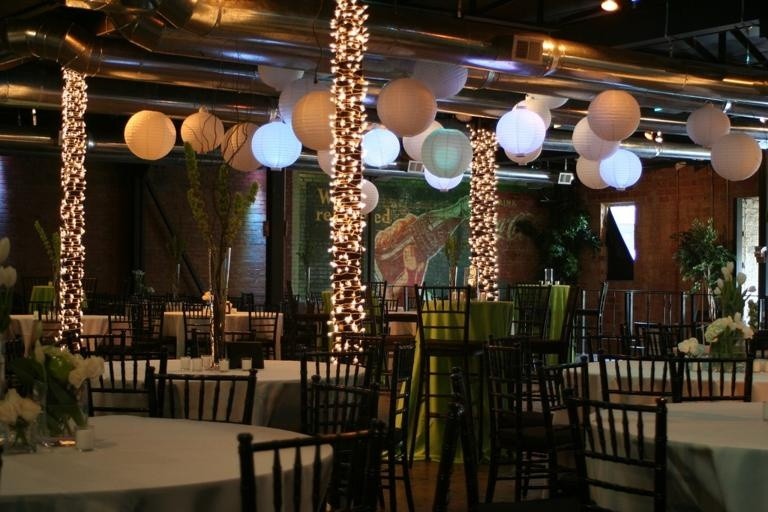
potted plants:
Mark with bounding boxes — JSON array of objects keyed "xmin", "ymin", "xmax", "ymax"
[
  {"xmin": 441, "ymin": 203, "xmax": 470, "ymax": 300},
  {"xmin": 183, "ymin": 142, "xmax": 258, "ymax": 370},
  {"xmin": 34, "ymin": 221, "xmax": 60, "ymax": 286},
  {"xmin": 669, "ymin": 217, "xmax": 736, "ymax": 320}
]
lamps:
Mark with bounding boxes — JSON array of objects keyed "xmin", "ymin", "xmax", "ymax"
[
  {"xmin": 250, "ymin": 117, "xmax": 303, "ymax": 173},
  {"xmin": 289, "ymin": 88, "xmax": 335, "ymax": 153},
  {"xmin": 221, "ymin": 120, "xmax": 262, "ymax": 172},
  {"xmin": 277, "ymin": 77, "xmax": 328, "ymax": 124},
  {"xmin": 422, "ymin": 169, "xmax": 465, "ymax": 193},
  {"xmin": 570, "ymin": 115, "xmax": 620, "ymax": 161},
  {"xmin": 524, "ymin": 92, "xmax": 568, "ymax": 110},
  {"xmin": 420, "ymin": 127, "xmax": 473, "ymax": 179},
  {"xmin": 411, "ymin": 62, "xmax": 469, "ymax": 103},
  {"xmin": 597, "ymin": 148, "xmax": 643, "ymax": 192},
  {"xmin": 402, "ymin": 119, "xmax": 444, "ymax": 161},
  {"xmin": 503, "ymin": 146, "xmax": 543, "ymax": 165},
  {"xmin": 511, "ymin": 100, "xmax": 553, "ymax": 131},
  {"xmin": 495, "ymin": 105, "xmax": 547, "ymax": 158},
  {"xmin": 123, "ymin": 107, "xmax": 179, "ymax": 163},
  {"xmin": 374, "ymin": 77, "xmax": 439, "ymax": 138},
  {"xmin": 178, "ymin": 103, "xmax": 226, "ymax": 156},
  {"xmin": 575, "ymin": 155, "xmax": 608, "ymax": 190},
  {"xmin": 684, "ymin": 102, "xmax": 731, "ymax": 149},
  {"xmin": 362, "ymin": 127, "xmax": 402, "ymax": 168},
  {"xmin": 585, "ymin": 88, "xmax": 642, "ymax": 143}
]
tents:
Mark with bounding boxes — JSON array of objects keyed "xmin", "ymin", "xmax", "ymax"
[{"xmin": 687, "ymin": 105, "xmax": 732, "ymax": 146}]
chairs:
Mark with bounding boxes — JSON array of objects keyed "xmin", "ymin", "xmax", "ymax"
[{"xmin": 4, "ymin": 282, "xmax": 766, "ymax": 512}]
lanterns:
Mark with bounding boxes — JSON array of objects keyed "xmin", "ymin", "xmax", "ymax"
[
  {"xmin": 125, "ymin": 110, "xmax": 176, "ymax": 162},
  {"xmin": 179, "ymin": 63, "xmax": 645, "ymax": 191}
]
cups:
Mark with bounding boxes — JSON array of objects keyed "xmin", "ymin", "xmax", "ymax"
[
  {"xmin": 73, "ymin": 424, "xmax": 94, "ymax": 450},
  {"xmin": 180, "ymin": 355, "xmax": 252, "ymax": 372},
  {"xmin": 545, "ymin": 267, "xmax": 553, "ymax": 287}
]
